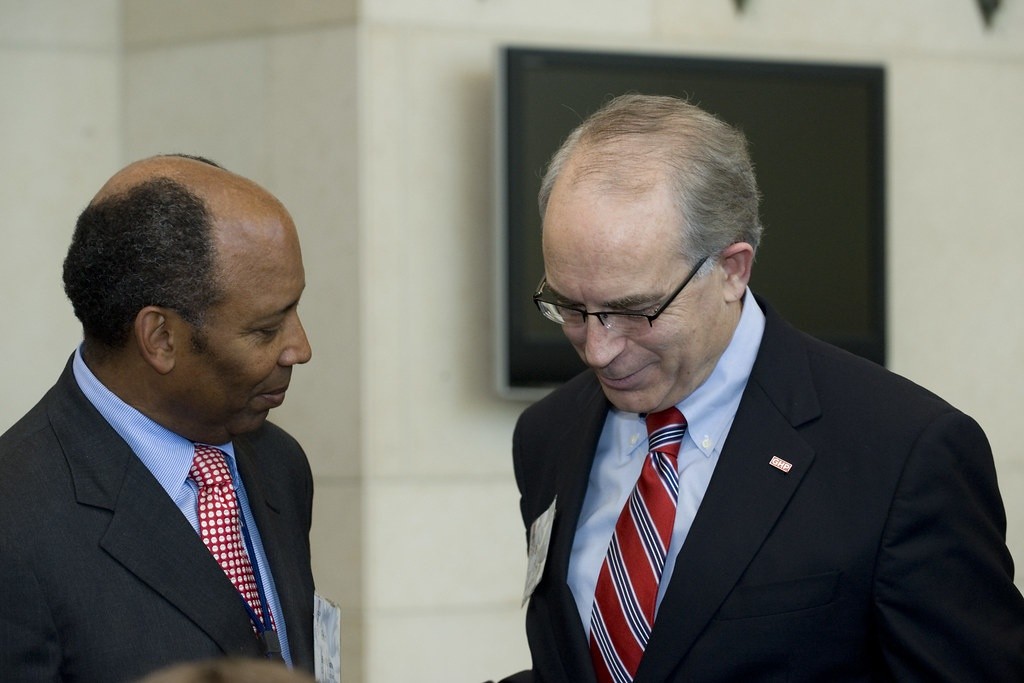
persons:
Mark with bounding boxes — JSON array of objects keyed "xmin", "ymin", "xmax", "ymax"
[
  {"xmin": 490, "ymin": 90, "xmax": 1023, "ymax": 683},
  {"xmin": 0, "ymin": 152, "xmax": 317, "ymax": 683}
]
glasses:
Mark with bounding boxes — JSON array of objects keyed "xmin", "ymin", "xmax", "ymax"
[{"xmin": 531, "ymin": 253, "xmax": 712, "ymax": 335}]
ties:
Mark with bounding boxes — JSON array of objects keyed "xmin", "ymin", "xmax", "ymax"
[
  {"xmin": 188, "ymin": 444, "xmax": 280, "ymax": 642},
  {"xmin": 589, "ymin": 407, "xmax": 688, "ymax": 683}
]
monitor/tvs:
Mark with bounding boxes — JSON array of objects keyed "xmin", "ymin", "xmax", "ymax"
[{"xmin": 490, "ymin": 43, "xmax": 889, "ymax": 401}]
